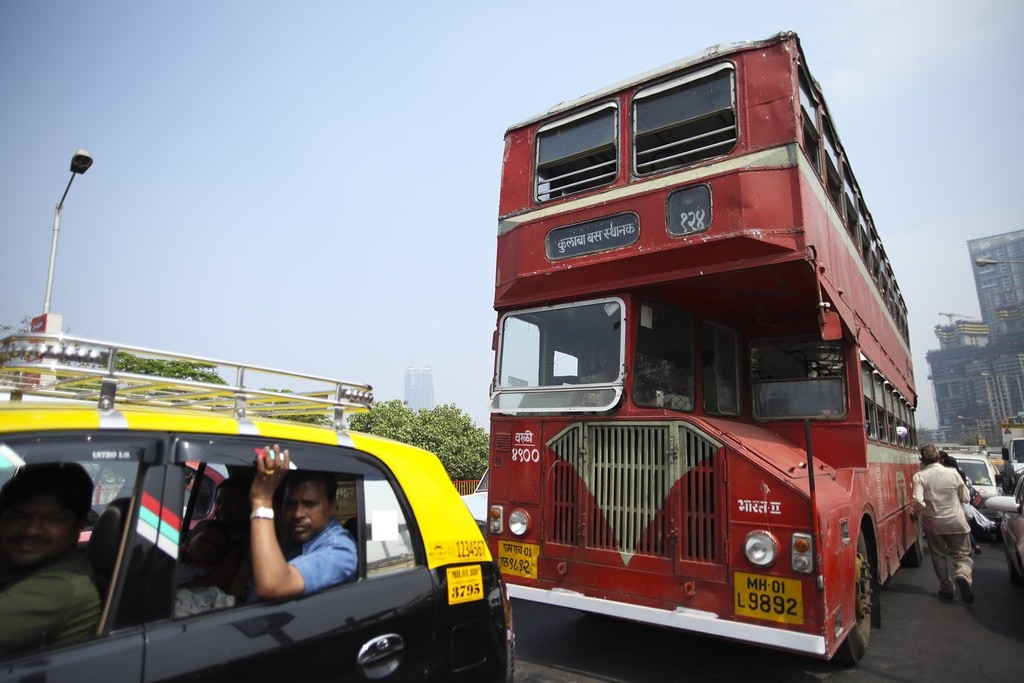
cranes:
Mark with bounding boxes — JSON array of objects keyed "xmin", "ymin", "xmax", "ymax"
[{"xmin": 938, "ymin": 312, "xmax": 975, "ymax": 325}]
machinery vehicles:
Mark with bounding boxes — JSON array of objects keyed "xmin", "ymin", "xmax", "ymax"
[{"xmin": 486, "ymin": 31, "xmax": 923, "ymax": 669}]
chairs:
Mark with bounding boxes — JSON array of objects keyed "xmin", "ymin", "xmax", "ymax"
[{"xmin": 84, "ymin": 497, "xmax": 130, "ymax": 604}]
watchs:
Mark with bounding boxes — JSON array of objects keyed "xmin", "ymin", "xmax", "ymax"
[{"xmin": 250, "ymin": 506, "xmax": 274, "ymax": 520}]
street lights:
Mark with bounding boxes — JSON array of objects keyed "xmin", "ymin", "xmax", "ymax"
[
  {"xmin": 44, "ymin": 148, "xmax": 94, "ymax": 315},
  {"xmin": 980, "ymin": 372, "xmax": 1024, "ymax": 410},
  {"xmin": 958, "ymin": 416, "xmax": 981, "ymax": 436}
]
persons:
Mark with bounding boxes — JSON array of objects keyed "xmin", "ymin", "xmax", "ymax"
[
  {"xmin": 0, "ymin": 461, "xmax": 101, "ymax": 657},
  {"xmin": 909, "ymin": 445, "xmax": 973, "ymax": 604},
  {"xmin": 939, "ymin": 451, "xmax": 990, "ymax": 556},
  {"xmin": 897, "ymin": 427, "xmax": 907, "ymax": 437},
  {"xmin": 173, "ymin": 444, "xmax": 357, "ymax": 617},
  {"xmin": 581, "ymin": 346, "xmax": 618, "ymax": 383}
]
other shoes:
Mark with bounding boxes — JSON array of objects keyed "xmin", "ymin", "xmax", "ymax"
[
  {"xmin": 939, "ymin": 590, "xmax": 953, "ymax": 598},
  {"xmin": 954, "ymin": 573, "xmax": 975, "ymax": 604},
  {"xmin": 975, "ymin": 545, "xmax": 981, "ymax": 555}
]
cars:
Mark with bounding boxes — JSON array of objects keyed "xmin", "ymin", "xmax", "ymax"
[
  {"xmin": 1002, "ymin": 438, "xmax": 1024, "ymax": 496},
  {"xmin": 984, "ymin": 472, "xmax": 1024, "ymax": 585},
  {"xmin": 947, "ymin": 453, "xmax": 1005, "ymax": 543},
  {"xmin": 0, "ymin": 334, "xmax": 516, "ymax": 683}
]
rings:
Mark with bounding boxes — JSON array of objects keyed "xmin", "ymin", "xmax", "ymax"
[{"xmin": 266, "ymin": 470, "xmax": 275, "ymax": 475}]
